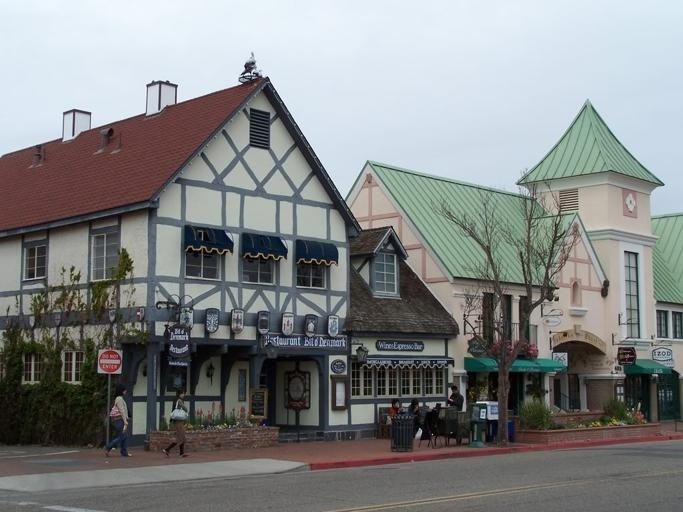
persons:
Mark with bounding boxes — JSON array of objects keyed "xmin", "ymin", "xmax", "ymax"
[
  {"xmin": 101, "ymin": 386, "xmax": 133, "ymax": 458},
  {"xmin": 447, "ymin": 386, "xmax": 463, "ymax": 410},
  {"xmin": 408, "ymin": 398, "xmax": 421, "ymax": 438},
  {"xmin": 389, "ymin": 398, "xmax": 402, "ymax": 417},
  {"xmin": 160, "ymin": 388, "xmax": 193, "ymax": 458}
]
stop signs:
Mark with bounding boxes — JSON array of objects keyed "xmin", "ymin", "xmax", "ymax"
[{"xmin": 97, "ymin": 350, "xmax": 122, "ymax": 374}]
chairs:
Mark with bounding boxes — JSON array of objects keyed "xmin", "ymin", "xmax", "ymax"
[{"xmin": 380, "ymin": 407, "xmax": 475, "ymax": 447}]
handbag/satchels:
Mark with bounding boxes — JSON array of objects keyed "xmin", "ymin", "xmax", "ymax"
[
  {"xmin": 109, "ymin": 397, "xmax": 125, "ymax": 417},
  {"xmin": 170, "ymin": 397, "xmax": 188, "ymax": 421}
]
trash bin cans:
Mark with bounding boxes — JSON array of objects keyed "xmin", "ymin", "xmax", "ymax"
[{"xmin": 391, "ymin": 414, "xmax": 418, "ymax": 452}]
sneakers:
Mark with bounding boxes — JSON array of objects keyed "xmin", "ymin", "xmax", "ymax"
[
  {"xmin": 162, "ymin": 446, "xmax": 191, "ymax": 459},
  {"xmin": 102, "ymin": 445, "xmax": 133, "ymax": 458}
]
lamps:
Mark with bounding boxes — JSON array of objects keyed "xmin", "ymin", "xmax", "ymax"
[
  {"xmin": 143, "ymin": 364, "xmax": 147, "ymax": 377},
  {"xmin": 550, "ymin": 331, "xmax": 567, "ymax": 337},
  {"xmin": 206, "ymin": 361, "xmax": 214, "ymax": 385},
  {"xmin": 350, "ymin": 341, "xmax": 367, "ymax": 364}
]
